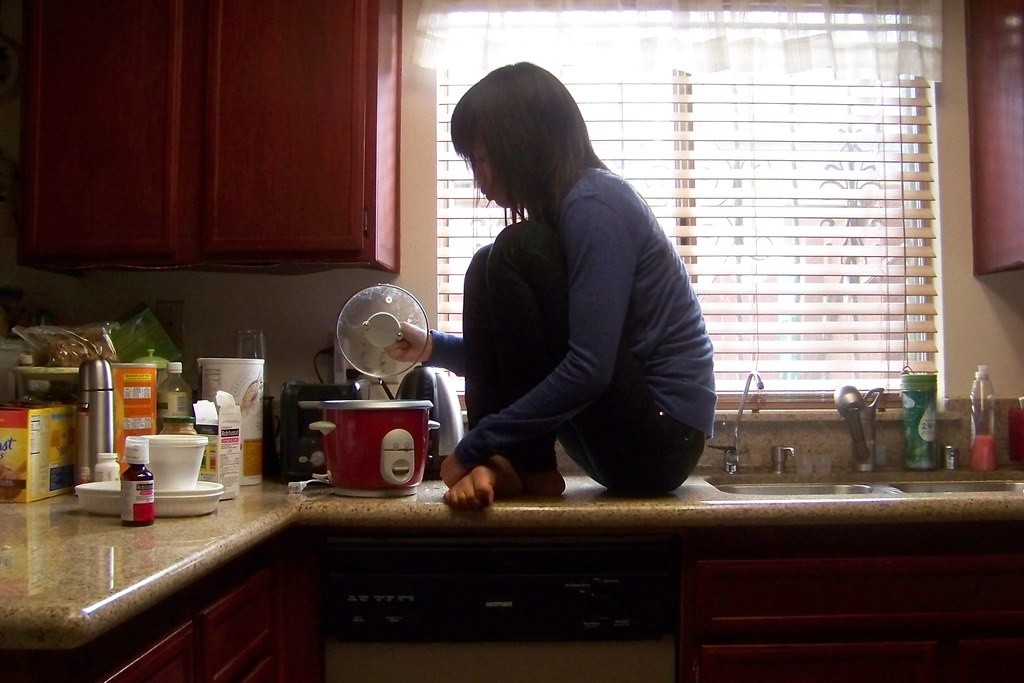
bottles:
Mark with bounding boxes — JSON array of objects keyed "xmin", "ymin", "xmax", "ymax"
[
  {"xmin": 157, "ymin": 363, "xmax": 190, "ymax": 414},
  {"xmin": 94, "ymin": 452, "xmax": 120, "ymax": 482},
  {"xmin": 901, "ymin": 371, "xmax": 939, "ymax": 470},
  {"xmin": 970, "ymin": 365, "xmax": 996, "ymax": 470},
  {"xmin": 17, "ymin": 352, "xmax": 34, "ymax": 366},
  {"xmin": 157, "ymin": 416, "xmax": 197, "ymax": 435},
  {"xmin": 122, "ymin": 436, "xmax": 155, "ymax": 526}
]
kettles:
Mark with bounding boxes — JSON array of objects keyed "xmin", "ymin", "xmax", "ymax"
[{"xmin": 394, "ymin": 365, "xmax": 464, "ymax": 477}]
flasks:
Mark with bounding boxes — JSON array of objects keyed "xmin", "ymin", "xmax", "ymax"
[{"xmin": 73, "ymin": 359, "xmax": 114, "ymax": 484}]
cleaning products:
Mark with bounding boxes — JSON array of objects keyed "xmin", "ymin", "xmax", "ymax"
[{"xmin": 970, "ymin": 365, "xmax": 995, "ymax": 471}]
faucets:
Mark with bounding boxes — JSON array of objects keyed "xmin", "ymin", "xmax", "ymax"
[
  {"xmin": 708, "ymin": 373, "xmax": 764, "ymax": 477},
  {"xmin": 835, "ymin": 386, "xmax": 886, "ymax": 473}
]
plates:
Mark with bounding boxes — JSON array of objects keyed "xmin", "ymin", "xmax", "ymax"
[{"xmin": 75, "ymin": 480, "xmax": 223, "ymax": 518}]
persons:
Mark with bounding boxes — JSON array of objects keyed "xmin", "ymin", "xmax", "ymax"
[{"xmin": 380, "ymin": 61, "xmax": 717, "ymax": 515}]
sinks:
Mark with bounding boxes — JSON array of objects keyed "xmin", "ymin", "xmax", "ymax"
[
  {"xmin": 890, "ymin": 480, "xmax": 1023, "ymax": 498},
  {"xmin": 705, "ymin": 472, "xmax": 891, "ymax": 506}
]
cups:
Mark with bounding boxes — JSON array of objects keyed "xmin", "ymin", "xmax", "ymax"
[
  {"xmin": 1008, "ymin": 407, "xmax": 1024, "ymax": 461},
  {"xmin": 236, "ymin": 328, "xmax": 269, "ymax": 393},
  {"xmin": 131, "ymin": 348, "xmax": 172, "ymax": 390}
]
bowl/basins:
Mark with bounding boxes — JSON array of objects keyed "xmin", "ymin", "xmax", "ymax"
[
  {"xmin": 135, "ymin": 434, "xmax": 210, "ymax": 491},
  {"xmin": 13, "ymin": 366, "xmax": 79, "ymax": 404}
]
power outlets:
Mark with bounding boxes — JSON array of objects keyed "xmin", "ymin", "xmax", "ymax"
[
  {"xmin": 326, "ymin": 332, "xmax": 400, "ymax": 384},
  {"xmin": 155, "ymin": 299, "xmax": 187, "ymax": 349}
]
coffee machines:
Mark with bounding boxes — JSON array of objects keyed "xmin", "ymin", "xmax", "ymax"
[{"xmin": 276, "ymin": 382, "xmax": 357, "ymax": 479}]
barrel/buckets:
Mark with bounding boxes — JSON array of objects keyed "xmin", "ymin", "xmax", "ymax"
[{"xmin": 197, "ymin": 355, "xmax": 265, "ymax": 485}]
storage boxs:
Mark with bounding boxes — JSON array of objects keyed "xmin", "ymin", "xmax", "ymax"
[
  {"xmin": 192, "ymin": 390, "xmax": 242, "ymax": 501},
  {"xmin": 0, "ymin": 405, "xmax": 78, "ymax": 504}
]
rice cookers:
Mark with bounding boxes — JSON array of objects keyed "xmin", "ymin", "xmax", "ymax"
[{"xmin": 310, "ymin": 399, "xmax": 439, "ymax": 498}]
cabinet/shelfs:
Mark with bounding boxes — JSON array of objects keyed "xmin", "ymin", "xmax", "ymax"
[
  {"xmin": 675, "ymin": 527, "xmax": 1024, "ymax": 683},
  {"xmin": 101, "ymin": 545, "xmax": 323, "ymax": 683},
  {"xmin": 964, "ymin": 0, "xmax": 1024, "ymax": 277},
  {"xmin": 15, "ymin": 0, "xmax": 404, "ymax": 273}
]
